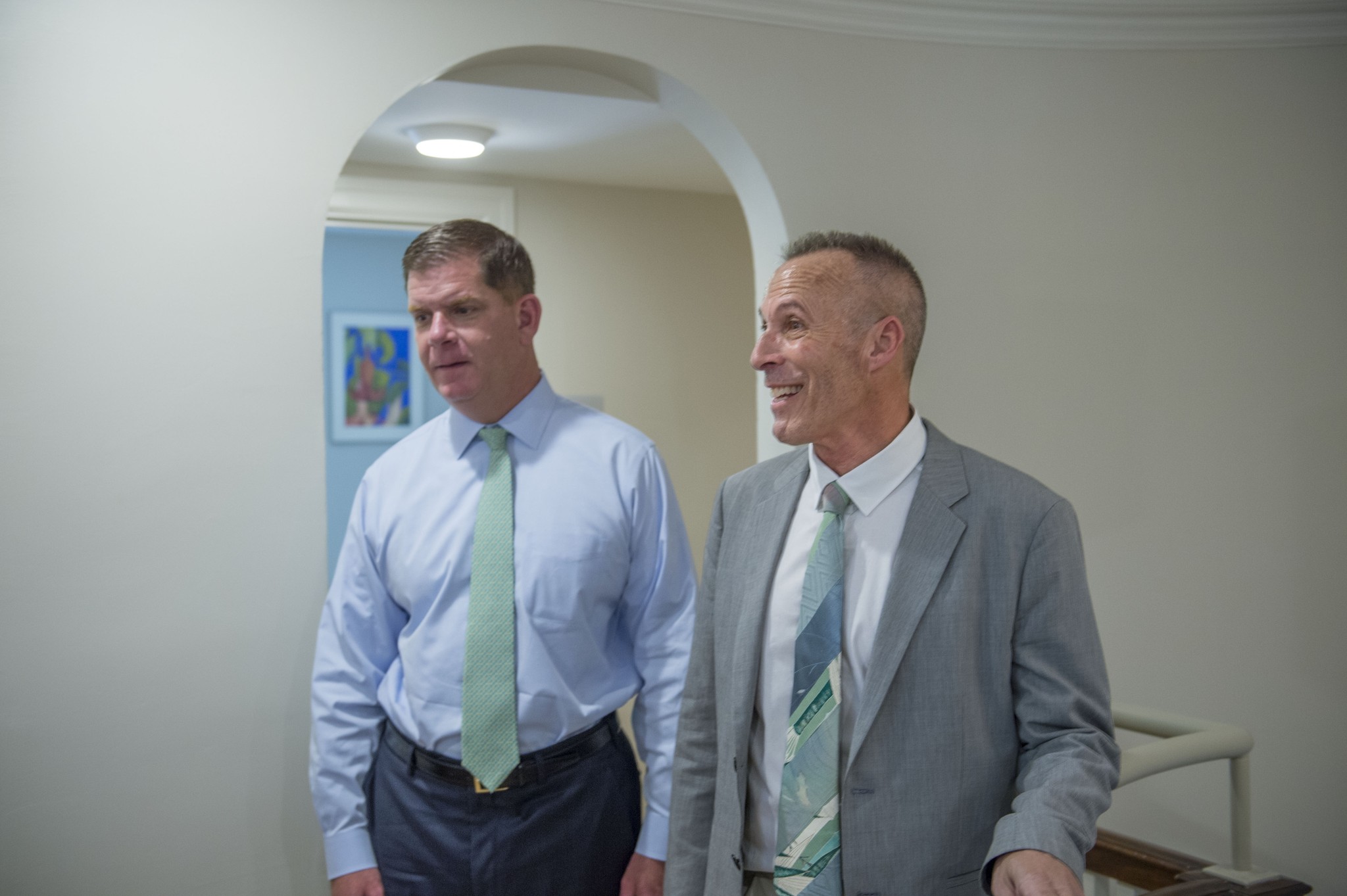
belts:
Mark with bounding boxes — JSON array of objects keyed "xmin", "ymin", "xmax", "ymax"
[{"xmin": 383, "ymin": 725, "xmax": 617, "ymax": 796}]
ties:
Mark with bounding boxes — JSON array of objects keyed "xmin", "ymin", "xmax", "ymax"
[
  {"xmin": 774, "ymin": 481, "xmax": 850, "ymax": 896},
  {"xmin": 461, "ymin": 425, "xmax": 521, "ymax": 792}
]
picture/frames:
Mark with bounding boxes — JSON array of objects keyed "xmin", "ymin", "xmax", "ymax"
[{"xmin": 329, "ymin": 309, "xmax": 424, "ymax": 444}]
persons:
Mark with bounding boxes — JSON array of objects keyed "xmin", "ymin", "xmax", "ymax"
[
  {"xmin": 666, "ymin": 232, "xmax": 1123, "ymax": 896},
  {"xmin": 306, "ymin": 219, "xmax": 697, "ymax": 895}
]
